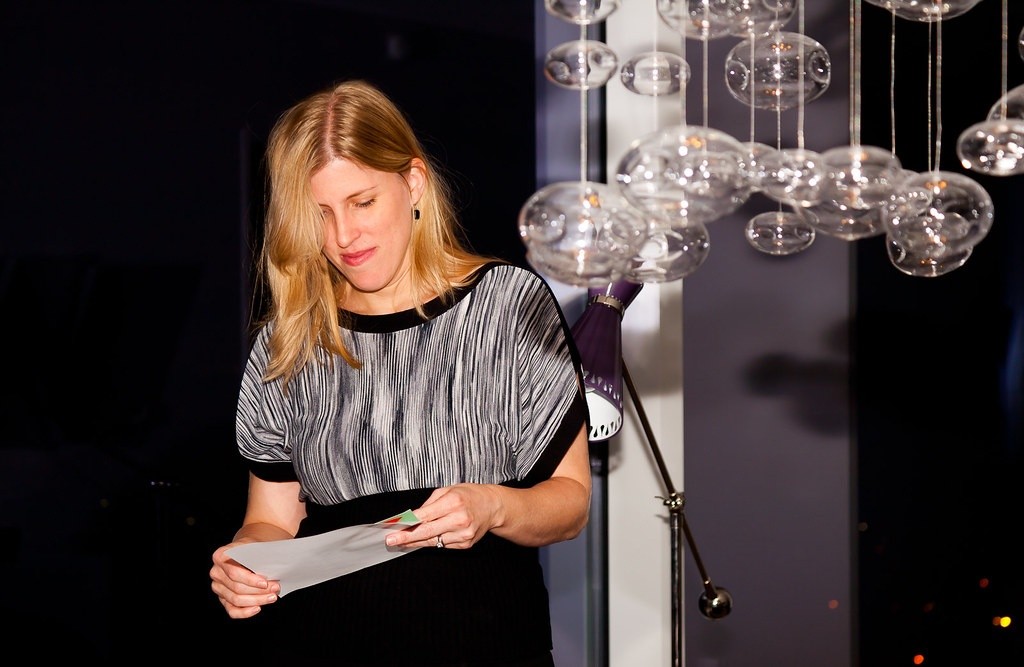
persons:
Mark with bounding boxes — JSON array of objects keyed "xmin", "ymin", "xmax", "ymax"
[{"xmin": 208, "ymin": 79, "xmax": 593, "ymax": 667}]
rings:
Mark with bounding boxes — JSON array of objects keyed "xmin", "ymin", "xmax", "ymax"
[{"xmin": 436, "ymin": 534, "xmax": 444, "ymax": 548}]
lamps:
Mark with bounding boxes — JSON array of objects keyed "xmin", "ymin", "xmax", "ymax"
[{"xmin": 568, "ymin": 257, "xmax": 732, "ymax": 666}]
